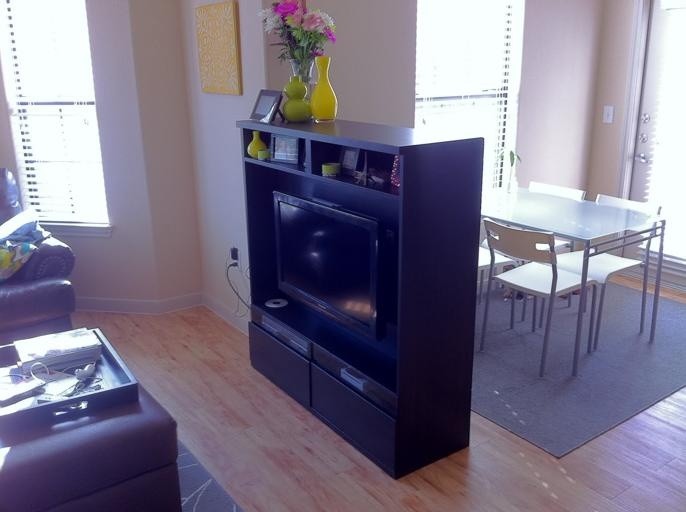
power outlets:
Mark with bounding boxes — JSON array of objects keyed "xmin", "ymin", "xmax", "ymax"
[{"xmin": 230, "ymin": 248, "xmax": 240, "ymax": 269}]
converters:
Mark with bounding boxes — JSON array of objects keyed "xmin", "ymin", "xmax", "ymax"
[{"xmin": 231, "ymin": 248, "xmax": 237, "ymax": 259}]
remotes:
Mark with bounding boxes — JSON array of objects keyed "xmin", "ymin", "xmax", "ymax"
[{"xmin": 0, "ymin": 379, "xmax": 46, "ymax": 407}]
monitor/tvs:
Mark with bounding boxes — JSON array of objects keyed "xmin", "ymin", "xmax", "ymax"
[{"xmin": 272, "ymin": 190, "xmax": 381, "ymax": 344}]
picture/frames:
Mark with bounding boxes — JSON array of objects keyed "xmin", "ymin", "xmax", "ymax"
[
  {"xmin": 339, "ymin": 146, "xmax": 360, "ymax": 176},
  {"xmin": 249, "ymin": 89, "xmax": 284, "ymax": 123},
  {"xmin": 269, "ymin": 134, "xmax": 300, "ymax": 165}
]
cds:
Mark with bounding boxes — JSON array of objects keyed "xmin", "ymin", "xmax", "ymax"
[{"xmin": 265, "ymin": 298, "xmax": 288, "ymax": 308}]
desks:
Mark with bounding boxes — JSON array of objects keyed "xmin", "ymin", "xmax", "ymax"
[{"xmin": 0, "ymin": 380, "xmax": 179, "ymax": 511}]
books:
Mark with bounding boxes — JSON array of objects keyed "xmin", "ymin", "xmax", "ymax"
[{"xmin": 13, "ymin": 326, "xmax": 102, "ymax": 375}]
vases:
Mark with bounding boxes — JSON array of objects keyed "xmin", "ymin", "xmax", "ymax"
[
  {"xmin": 283, "ymin": 53, "xmax": 338, "ymax": 125},
  {"xmin": 247, "ymin": 131, "xmax": 267, "ymax": 158}
]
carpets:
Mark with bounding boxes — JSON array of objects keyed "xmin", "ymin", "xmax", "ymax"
[
  {"xmin": 176, "ymin": 436, "xmax": 242, "ymax": 511},
  {"xmin": 471, "ymin": 271, "xmax": 686, "ymax": 458}
]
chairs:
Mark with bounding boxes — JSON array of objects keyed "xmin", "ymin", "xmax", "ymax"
[
  {"xmin": 0, "ymin": 169, "xmax": 75, "ymax": 346},
  {"xmin": 479, "ymin": 180, "xmax": 666, "ymax": 380}
]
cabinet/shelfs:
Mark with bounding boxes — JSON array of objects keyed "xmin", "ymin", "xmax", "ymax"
[{"xmin": 236, "ymin": 119, "xmax": 485, "ymax": 478}]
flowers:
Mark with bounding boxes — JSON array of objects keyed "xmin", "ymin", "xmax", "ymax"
[{"xmin": 260, "ymin": 0, "xmax": 337, "ymax": 75}]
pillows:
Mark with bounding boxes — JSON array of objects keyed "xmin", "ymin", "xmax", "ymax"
[{"xmin": 0, "ymin": 209, "xmax": 43, "ymax": 280}]
